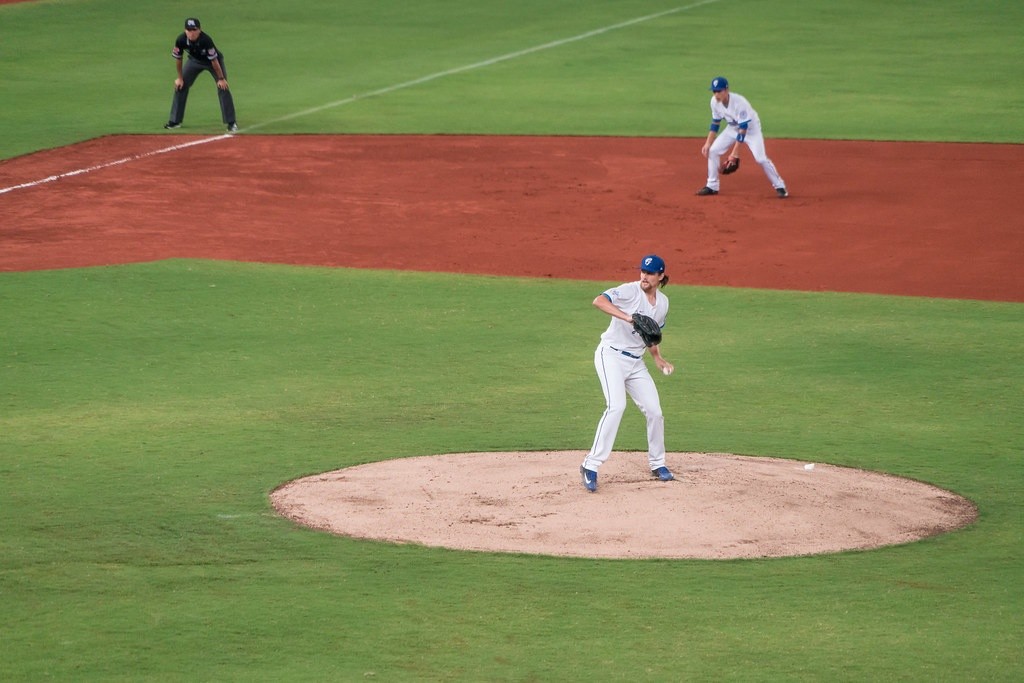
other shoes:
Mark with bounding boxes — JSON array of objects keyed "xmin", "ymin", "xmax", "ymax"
[
  {"xmin": 777, "ymin": 188, "xmax": 788, "ymax": 197},
  {"xmin": 164, "ymin": 120, "xmax": 182, "ymax": 129},
  {"xmin": 228, "ymin": 123, "xmax": 238, "ymax": 131},
  {"xmin": 696, "ymin": 187, "xmax": 718, "ymax": 195}
]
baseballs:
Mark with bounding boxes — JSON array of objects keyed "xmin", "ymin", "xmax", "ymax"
[{"xmin": 663, "ymin": 365, "xmax": 674, "ymax": 374}]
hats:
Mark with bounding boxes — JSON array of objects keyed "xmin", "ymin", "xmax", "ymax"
[
  {"xmin": 710, "ymin": 77, "xmax": 728, "ymax": 91},
  {"xmin": 641, "ymin": 255, "xmax": 665, "ymax": 273},
  {"xmin": 184, "ymin": 17, "xmax": 200, "ymax": 30}
]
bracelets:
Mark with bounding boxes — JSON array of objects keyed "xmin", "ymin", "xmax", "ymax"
[
  {"xmin": 736, "ymin": 132, "xmax": 746, "ymax": 143},
  {"xmin": 218, "ymin": 77, "xmax": 225, "ymax": 80},
  {"xmin": 710, "ymin": 124, "xmax": 720, "ymax": 133}
]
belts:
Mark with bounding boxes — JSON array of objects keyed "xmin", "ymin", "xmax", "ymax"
[{"xmin": 610, "ymin": 346, "xmax": 640, "ymax": 359}]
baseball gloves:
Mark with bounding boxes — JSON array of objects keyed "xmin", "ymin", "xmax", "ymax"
[
  {"xmin": 719, "ymin": 157, "xmax": 739, "ymax": 176},
  {"xmin": 632, "ymin": 314, "xmax": 662, "ymax": 347}
]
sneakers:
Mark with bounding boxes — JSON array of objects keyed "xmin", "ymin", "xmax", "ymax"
[
  {"xmin": 652, "ymin": 467, "xmax": 673, "ymax": 481},
  {"xmin": 580, "ymin": 465, "xmax": 597, "ymax": 491}
]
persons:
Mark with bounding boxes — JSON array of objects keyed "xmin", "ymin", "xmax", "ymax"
[
  {"xmin": 694, "ymin": 75, "xmax": 789, "ymax": 199},
  {"xmin": 580, "ymin": 254, "xmax": 676, "ymax": 491},
  {"xmin": 162, "ymin": 18, "xmax": 240, "ymax": 135}
]
